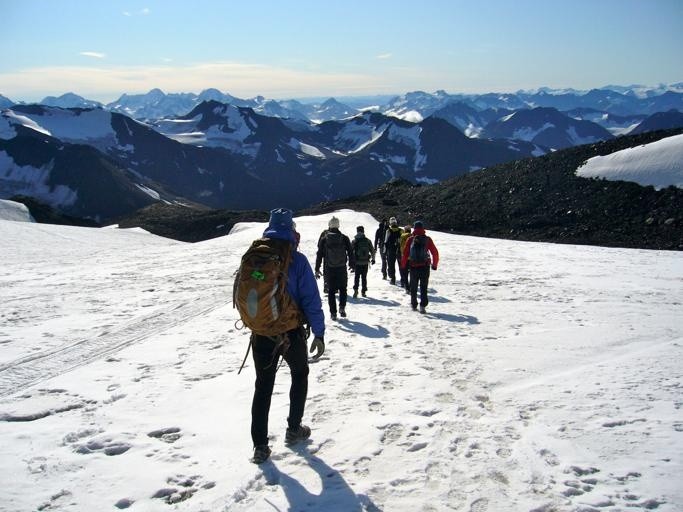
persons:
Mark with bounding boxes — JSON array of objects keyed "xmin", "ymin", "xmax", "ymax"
[
  {"xmin": 314, "ymin": 217, "xmax": 353, "ymax": 320},
  {"xmin": 349, "ymin": 226, "xmax": 375, "ymax": 300},
  {"xmin": 318, "ymin": 228, "xmax": 329, "ymax": 292},
  {"xmin": 374, "ymin": 216, "xmax": 438, "ymax": 315},
  {"xmin": 232, "ymin": 209, "xmax": 325, "ymax": 462}
]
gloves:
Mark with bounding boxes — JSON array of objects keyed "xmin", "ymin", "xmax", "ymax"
[
  {"xmin": 370, "ymin": 259, "xmax": 375, "ymax": 265},
  {"xmin": 348, "ymin": 264, "xmax": 355, "ymax": 273},
  {"xmin": 382, "ymin": 276, "xmax": 411, "ymax": 294},
  {"xmin": 309, "ymin": 337, "xmax": 326, "ymax": 360},
  {"xmin": 431, "ymin": 266, "xmax": 437, "ymax": 271},
  {"xmin": 314, "ymin": 270, "xmax": 322, "ymax": 279}
]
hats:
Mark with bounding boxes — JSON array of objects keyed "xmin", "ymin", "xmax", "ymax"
[
  {"xmin": 356, "ymin": 226, "xmax": 363, "ymax": 234},
  {"xmin": 413, "ymin": 221, "xmax": 424, "ymax": 230},
  {"xmin": 328, "ymin": 215, "xmax": 340, "ymax": 228},
  {"xmin": 268, "ymin": 208, "xmax": 294, "ymax": 230},
  {"xmin": 389, "ymin": 217, "xmax": 397, "ymax": 227}
]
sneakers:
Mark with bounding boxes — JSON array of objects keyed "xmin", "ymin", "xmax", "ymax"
[
  {"xmin": 352, "ymin": 291, "xmax": 368, "ymax": 298},
  {"xmin": 283, "ymin": 424, "xmax": 311, "ymax": 445},
  {"xmin": 411, "ymin": 305, "xmax": 426, "ymax": 314},
  {"xmin": 331, "ymin": 313, "xmax": 337, "ymax": 320},
  {"xmin": 251, "ymin": 446, "xmax": 271, "ymax": 464},
  {"xmin": 338, "ymin": 306, "xmax": 346, "ymax": 318}
]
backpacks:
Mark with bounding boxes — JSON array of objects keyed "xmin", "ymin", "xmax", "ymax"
[
  {"xmin": 324, "ymin": 232, "xmax": 347, "ymax": 269},
  {"xmin": 409, "ymin": 234, "xmax": 429, "ymax": 264},
  {"xmin": 230, "ymin": 238, "xmax": 304, "ymax": 337},
  {"xmin": 386, "ymin": 228, "xmax": 401, "ymax": 250},
  {"xmin": 353, "ymin": 238, "xmax": 370, "ymax": 261}
]
